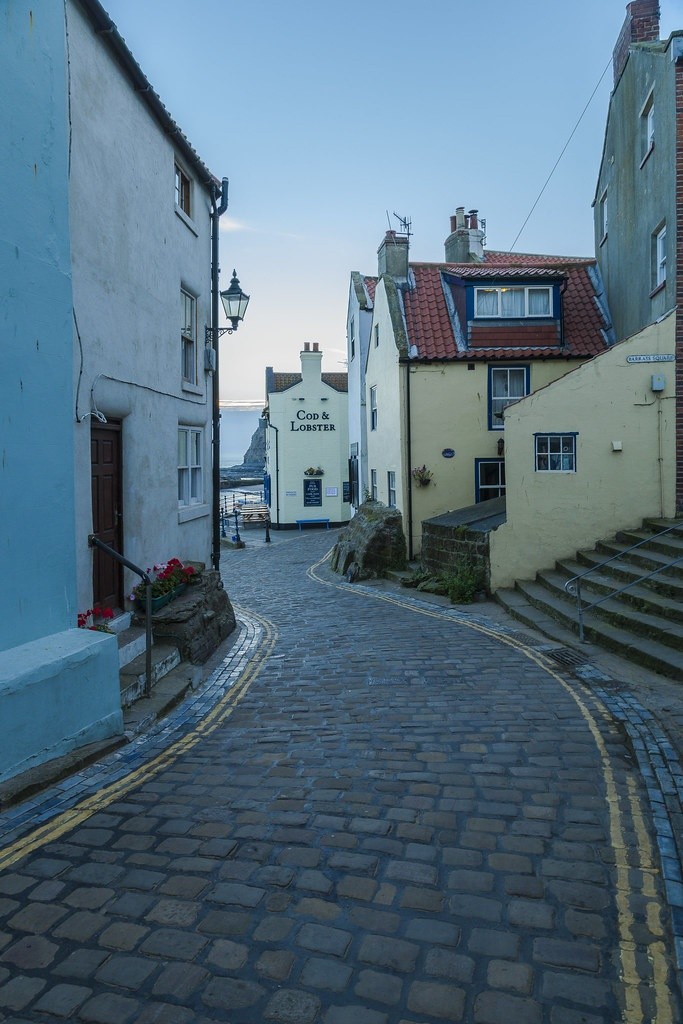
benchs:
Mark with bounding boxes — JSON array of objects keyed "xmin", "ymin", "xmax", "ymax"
[
  {"xmin": 240, "ymin": 516, "xmax": 271, "ymax": 529},
  {"xmin": 296, "ymin": 518, "xmax": 330, "ymax": 531}
]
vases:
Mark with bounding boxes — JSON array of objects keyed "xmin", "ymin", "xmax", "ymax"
[
  {"xmin": 141, "ymin": 590, "xmax": 171, "ymax": 611},
  {"xmin": 171, "ymin": 583, "xmax": 188, "ymax": 596},
  {"xmin": 419, "ymin": 479, "xmax": 430, "ymax": 485}
]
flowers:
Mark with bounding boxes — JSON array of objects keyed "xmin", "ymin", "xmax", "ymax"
[
  {"xmin": 127, "ymin": 557, "xmax": 195, "ymax": 603},
  {"xmin": 77, "ymin": 608, "xmax": 117, "ymax": 634},
  {"xmin": 411, "ymin": 465, "xmax": 433, "ymax": 482}
]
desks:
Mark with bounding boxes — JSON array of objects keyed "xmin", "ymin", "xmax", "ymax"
[{"xmin": 239, "ymin": 504, "xmax": 270, "ymax": 519}]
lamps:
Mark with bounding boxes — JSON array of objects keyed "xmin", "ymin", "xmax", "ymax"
[{"xmin": 204, "ymin": 270, "xmax": 251, "ymax": 343}]
